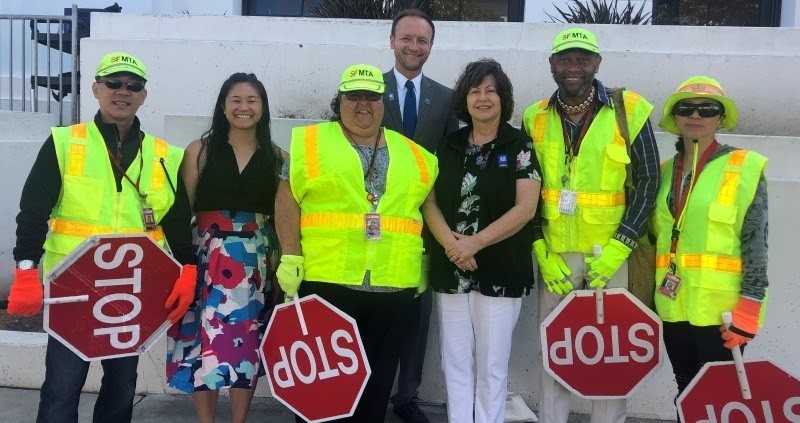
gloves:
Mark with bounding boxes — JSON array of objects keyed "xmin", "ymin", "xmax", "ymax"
[
  {"xmin": 584, "ymin": 237, "xmax": 632, "ymax": 289},
  {"xmin": 720, "ymin": 296, "xmax": 761, "ymax": 350},
  {"xmin": 164, "ymin": 264, "xmax": 198, "ymax": 327},
  {"xmin": 531, "ymin": 238, "xmax": 574, "ymax": 295},
  {"xmin": 276, "ymin": 255, "xmax": 306, "ymax": 299},
  {"xmin": 7, "ymin": 269, "xmax": 43, "ymax": 319}
]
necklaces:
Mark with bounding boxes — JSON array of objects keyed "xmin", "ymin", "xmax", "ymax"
[
  {"xmin": 471, "ymin": 129, "xmax": 498, "ymax": 157},
  {"xmin": 555, "ymin": 85, "xmax": 594, "ymax": 115},
  {"xmin": 339, "ymin": 119, "xmax": 382, "ymax": 201}
]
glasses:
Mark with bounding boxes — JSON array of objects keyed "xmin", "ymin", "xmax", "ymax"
[
  {"xmin": 674, "ymin": 102, "xmax": 722, "ymax": 118},
  {"xmin": 98, "ymin": 79, "xmax": 144, "ymax": 92},
  {"xmin": 343, "ymin": 92, "xmax": 384, "ymax": 102}
]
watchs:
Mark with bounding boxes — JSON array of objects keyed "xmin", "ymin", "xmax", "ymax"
[{"xmin": 16, "ymin": 260, "xmax": 39, "ymax": 271}]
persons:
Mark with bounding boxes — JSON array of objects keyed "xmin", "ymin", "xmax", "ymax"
[
  {"xmin": 379, "ymin": 9, "xmax": 461, "ymax": 423},
  {"xmin": 645, "ymin": 75, "xmax": 772, "ymax": 423},
  {"xmin": 5, "ymin": 53, "xmax": 198, "ymax": 422},
  {"xmin": 520, "ymin": 27, "xmax": 663, "ymax": 423},
  {"xmin": 181, "ymin": 72, "xmax": 287, "ymax": 423},
  {"xmin": 273, "ymin": 62, "xmax": 436, "ymax": 422},
  {"xmin": 419, "ymin": 56, "xmax": 542, "ymax": 423}
]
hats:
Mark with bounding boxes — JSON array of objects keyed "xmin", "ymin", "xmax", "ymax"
[
  {"xmin": 96, "ymin": 52, "xmax": 149, "ymax": 84},
  {"xmin": 551, "ymin": 27, "xmax": 600, "ymax": 58},
  {"xmin": 659, "ymin": 75, "xmax": 740, "ymax": 135},
  {"xmin": 339, "ymin": 64, "xmax": 385, "ymax": 93}
]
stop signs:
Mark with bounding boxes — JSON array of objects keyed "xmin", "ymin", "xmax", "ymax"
[
  {"xmin": 258, "ymin": 293, "xmax": 373, "ymax": 423},
  {"xmin": 43, "ymin": 233, "xmax": 186, "ymax": 362},
  {"xmin": 675, "ymin": 358, "xmax": 800, "ymax": 423},
  {"xmin": 539, "ymin": 287, "xmax": 664, "ymax": 401}
]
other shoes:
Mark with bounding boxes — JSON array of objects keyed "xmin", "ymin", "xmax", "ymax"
[{"xmin": 393, "ymin": 397, "xmax": 430, "ymax": 423}]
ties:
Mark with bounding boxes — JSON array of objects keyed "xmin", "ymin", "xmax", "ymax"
[{"xmin": 403, "ymin": 80, "xmax": 417, "ymax": 139}]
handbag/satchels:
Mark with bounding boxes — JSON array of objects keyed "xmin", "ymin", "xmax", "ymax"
[
  {"xmin": 503, "ymin": 392, "xmax": 538, "ymax": 422},
  {"xmin": 628, "ymin": 243, "xmax": 657, "ymax": 310}
]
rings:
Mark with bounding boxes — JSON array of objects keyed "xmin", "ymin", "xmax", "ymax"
[{"xmin": 453, "ymin": 256, "xmax": 458, "ymax": 260}]
